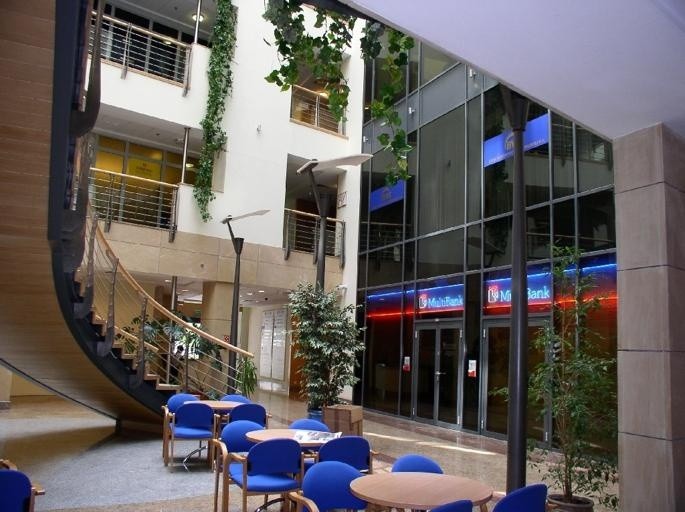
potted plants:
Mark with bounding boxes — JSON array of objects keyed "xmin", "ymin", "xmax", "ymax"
[
  {"xmin": 486, "ymin": 242, "xmax": 621, "ymax": 512},
  {"xmin": 286, "ymin": 280, "xmax": 366, "ymax": 420}
]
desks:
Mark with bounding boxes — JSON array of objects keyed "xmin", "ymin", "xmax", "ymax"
[
  {"xmin": 245, "ymin": 428, "xmax": 340, "ymax": 447},
  {"xmin": 350, "ymin": 472, "xmax": 494, "ymax": 512},
  {"xmin": 184, "ymin": 400, "xmax": 245, "ymax": 410}
]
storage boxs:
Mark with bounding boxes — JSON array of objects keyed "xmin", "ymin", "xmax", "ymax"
[{"xmin": 322, "ymin": 404, "xmax": 364, "ymax": 436}]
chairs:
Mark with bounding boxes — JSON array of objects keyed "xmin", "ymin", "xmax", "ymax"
[
  {"xmin": 223, "ymin": 404, "xmax": 273, "ymax": 429},
  {"xmin": 427, "ymin": 499, "xmax": 475, "ymax": 512},
  {"xmin": 212, "ymin": 420, "xmax": 264, "ymax": 470},
  {"xmin": 219, "ymin": 438, "xmax": 304, "ymax": 512},
  {"xmin": 221, "ymin": 394, "xmax": 254, "ymax": 404},
  {"xmin": 167, "ymin": 403, "xmax": 220, "ymax": 467},
  {"xmin": 490, "ymin": 484, "xmax": 547, "ymax": 512},
  {"xmin": 392, "ymin": 454, "xmax": 450, "ymax": 476},
  {"xmin": 1, "ymin": 470, "xmax": 46, "ymax": 512},
  {"xmin": 0, "ymin": 455, "xmax": 18, "ymax": 470},
  {"xmin": 161, "ymin": 393, "xmax": 200, "ymax": 415},
  {"xmin": 288, "ymin": 461, "xmax": 367, "ymax": 512},
  {"xmin": 304, "ymin": 437, "xmax": 380, "ymax": 475}
]
